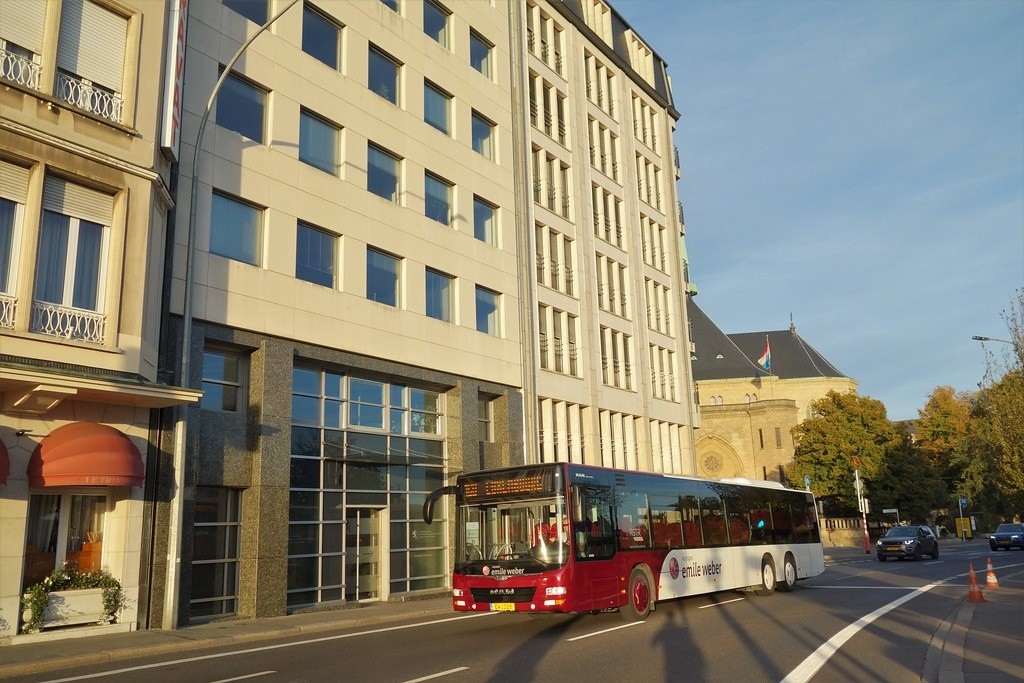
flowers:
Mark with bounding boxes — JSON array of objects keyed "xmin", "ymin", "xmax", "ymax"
[{"xmin": 25, "ymin": 569, "xmax": 121, "ymax": 630}]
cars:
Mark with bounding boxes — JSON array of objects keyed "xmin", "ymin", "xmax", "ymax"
[{"xmin": 989, "ymin": 523, "xmax": 1024, "ymax": 552}]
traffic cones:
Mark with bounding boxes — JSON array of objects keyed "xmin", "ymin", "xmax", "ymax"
[
  {"xmin": 967, "ymin": 563, "xmax": 989, "ymax": 602},
  {"xmin": 985, "ymin": 557, "xmax": 1002, "ymax": 590}
]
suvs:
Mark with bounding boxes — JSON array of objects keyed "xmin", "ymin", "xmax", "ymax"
[{"xmin": 876, "ymin": 526, "xmax": 938, "ymax": 562}]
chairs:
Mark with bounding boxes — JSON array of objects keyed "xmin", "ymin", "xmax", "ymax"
[{"xmin": 531, "ymin": 510, "xmax": 813, "ymax": 567}]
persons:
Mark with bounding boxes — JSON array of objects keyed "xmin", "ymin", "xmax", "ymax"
[{"xmin": 549, "ymin": 516, "xmax": 586, "ymax": 557}]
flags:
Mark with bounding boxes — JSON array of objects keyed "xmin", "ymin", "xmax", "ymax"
[{"xmin": 757, "ymin": 338, "xmax": 772, "ymax": 371}]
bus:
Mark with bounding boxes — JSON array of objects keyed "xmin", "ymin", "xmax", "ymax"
[{"xmin": 423, "ymin": 461, "xmax": 827, "ymax": 620}]
